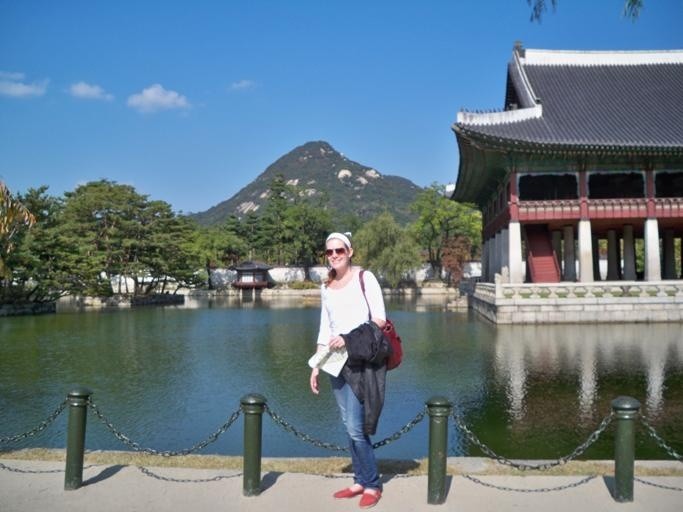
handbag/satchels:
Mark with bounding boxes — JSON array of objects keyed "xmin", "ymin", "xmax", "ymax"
[{"xmin": 358, "ymin": 270, "xmax": 401, "ymax": 370}]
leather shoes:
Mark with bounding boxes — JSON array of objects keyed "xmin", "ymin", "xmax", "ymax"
[
  {"xmin": 359, "ymin": 491, "xmax": 380, "ymax": 509},
  {"xmin": 333, "ymin": 488, "xmax": 363, "ymax": 498}
]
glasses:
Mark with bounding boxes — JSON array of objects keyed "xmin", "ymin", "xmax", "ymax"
[{"xmin": 325, "ymin": 248, "xmax": 344, "ymax": 256}]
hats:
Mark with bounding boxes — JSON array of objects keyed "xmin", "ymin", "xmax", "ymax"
[{"xmin": 325, "ymin": 231, "xmax": 352, "ymax": 249}]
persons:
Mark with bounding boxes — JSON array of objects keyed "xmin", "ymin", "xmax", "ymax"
[{"xmin": 308, "ymin": 232, "xmax": 386, "ymax": 509}]
